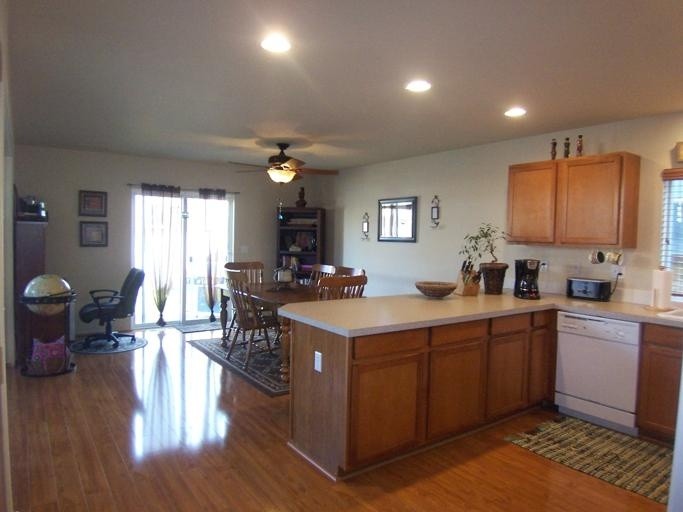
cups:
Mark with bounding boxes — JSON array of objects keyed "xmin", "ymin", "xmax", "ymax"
[{"xmin": 590, "ymin": 248, "xmax": 624, "ymax": 268}]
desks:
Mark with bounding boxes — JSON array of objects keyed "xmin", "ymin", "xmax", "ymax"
[{"xmin": 215, "ymin": 282, "xmax": 367, "ymax": 353}]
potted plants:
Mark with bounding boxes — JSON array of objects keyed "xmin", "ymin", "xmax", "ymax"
[{"xmin": 458, "ymin": 223, "xmax": 515, "ymax": 295}]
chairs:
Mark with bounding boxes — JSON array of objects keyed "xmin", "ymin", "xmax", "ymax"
[
  {"xmin": 79, "ymin": 267, "xmax": 145, "ymax": 350},
  {"xmin": 223, "ymin": 261, "xmax": 368, "ymax": 371}
]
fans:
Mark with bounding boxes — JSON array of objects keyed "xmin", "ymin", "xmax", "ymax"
[{"xmin": 223, "ymin": 154, "xmax": 340, "ymax": 186}]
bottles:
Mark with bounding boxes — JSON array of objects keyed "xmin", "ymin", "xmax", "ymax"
[{"xmin": 550, "ymin": 134, "xmax": 582, "ymax": 160}]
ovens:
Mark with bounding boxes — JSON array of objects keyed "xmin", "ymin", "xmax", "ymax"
[{"xmin": 554, "ymin": 310, "xmax": 641, "ymax": 440}]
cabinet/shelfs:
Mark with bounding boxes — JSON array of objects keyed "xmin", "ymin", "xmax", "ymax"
[
  {"xmin": 635, "ymin": 319, "xmax": 681, "ymax": 433},
  {"xmin": 505, "ymin": 149, "xmax": 639, "ymax": 249},
  {"xmin": 277, "ymin": 206, "xmax": 325, "ymax": 276}
]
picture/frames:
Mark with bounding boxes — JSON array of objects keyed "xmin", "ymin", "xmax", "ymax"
[{"xmin": 79, "ymin": 190, "xmax": 108, "ymax": 247}]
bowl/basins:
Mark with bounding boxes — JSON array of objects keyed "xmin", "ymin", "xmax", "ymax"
[{"xmin": 414, "ymin": 281, "xmax": 456, "ymax": 299}]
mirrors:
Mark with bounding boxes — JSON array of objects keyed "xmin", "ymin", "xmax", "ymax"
[{"xmin": 377, "ymin": 197, "xmax": 416, "ymax": 242}]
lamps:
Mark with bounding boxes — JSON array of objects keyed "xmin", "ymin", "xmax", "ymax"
[{"xmin": 269, "ymin": 154, "xmax": 295, "ymax": 185}]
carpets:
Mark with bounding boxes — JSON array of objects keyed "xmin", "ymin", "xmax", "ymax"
[
  {"xmin": 172, "ymin": 317, "xmax": 292, "ymax": 398},
  {"xmin": 68, "ymin": 336, "xmax": 148, "ymax": 356},
  {"xmin": 510, "ymin": 414, "xmax": 671, "ymax": 506}
]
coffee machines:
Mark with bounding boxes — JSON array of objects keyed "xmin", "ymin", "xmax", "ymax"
[{"xmin": 515, "ymin": 258, "xmax": 539, "ymax": 299}]
